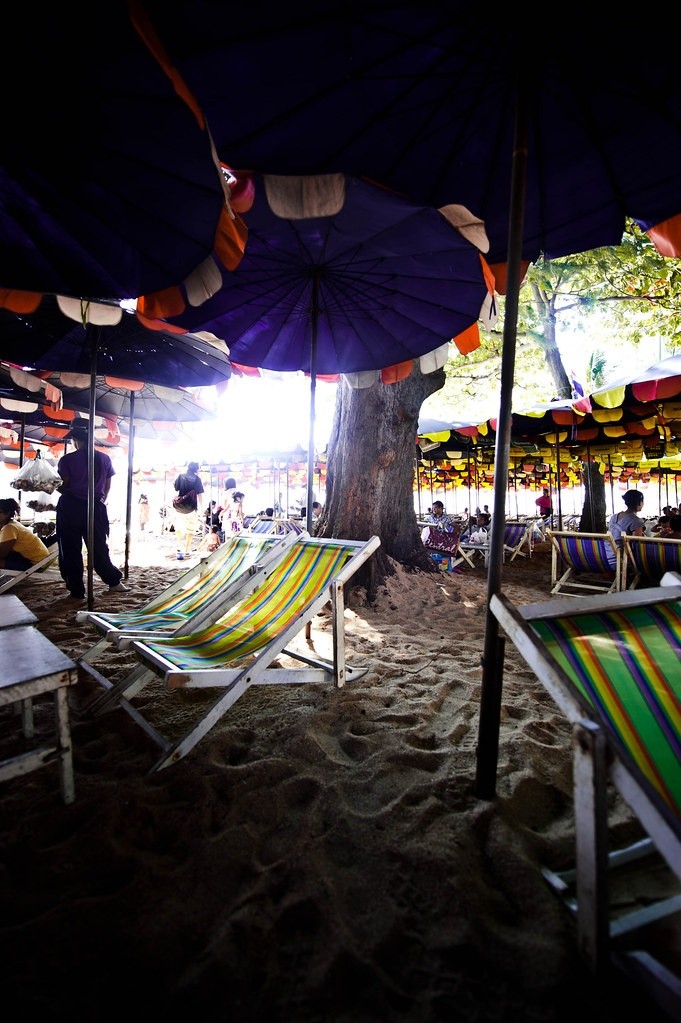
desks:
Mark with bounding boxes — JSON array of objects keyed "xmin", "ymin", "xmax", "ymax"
[
  {"xmin": 0, "ymin": 626, "xmax": 81, "ymax": 806},
  {"xmin": 0, "ymin": 594, "xmax": 40, "ymax": 719}
]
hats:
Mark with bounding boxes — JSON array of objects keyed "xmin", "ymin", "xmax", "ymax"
[{"xmin": 61, "ymin": 417, "xmax": 89, "ymax": 440}]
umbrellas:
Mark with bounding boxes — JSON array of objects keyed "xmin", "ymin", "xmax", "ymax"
[{"xmin": 0, "ymin": 0, "xmax": 681, "ymax": 796}]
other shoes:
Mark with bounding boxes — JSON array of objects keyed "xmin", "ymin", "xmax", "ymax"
[
  {"xmin": 110, "ymin": 582, "xmax": 132, "ymax": 592},
  {"xmin": 177, "ymin": 554, "xmax": 183, "ymax": 559}
]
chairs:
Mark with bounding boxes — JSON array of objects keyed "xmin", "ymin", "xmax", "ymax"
[{"xmin": 0, "ymin": 511, "xmax": 681, "ymax": 1023}]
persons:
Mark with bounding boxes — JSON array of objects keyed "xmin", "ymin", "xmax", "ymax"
[
  {"xmin": 220, "ymin": 477, "xmax": 240, "ymax": 532},
  {"xmin": 197, "ymin": 524, "xmax": 220, "ymax": 551},
  {"xmin": 138, "ymin": 494, "xmax": 149, "ymax": 530},
  {"xmin": 603, "ymin": 489, "xmax": 644, "ymax": 568},
  {"xmin": 458, "ymin": 507, "xmax": 468, "ymax": 520},
  {"xmin": 429, "ymin": 500, "xmax": 451, "ymax": 530},
  {"xmin": 459, "ymin": 514, "xmax": 490, "ymax": 543},
  {"xmin": 0, "ymin": 498, "xmax": 49, "ymax": 573},
  {"xmin": 172, "ymin": 460, "xmax": 204, "ymax": 561},
  {"xmin": 653, "ymin": 516, "xmax": 672, "ymax": 537},
  {"xmin": 297, "ymin": 484, "xmax": 316, "ymax": 506},
  {"xmin": 474, "ymin": 507, "xmax": 481, "ymax": 516},
  {"xmin": 209, "ymin": 505, "xmax": 223, "ymax": 534},
  {"xmin": 301, "ymin": 502, "xmax": 322, "ymax": 526},
  {"xmin": 665, "ymin": 515, "xmax": 681, "ymax": 539},
  {"xmin": 266, "ymin": 507, "xmax": 273, "ymax": 517},
  {"xmin": 204, "ymin": 501, "xmax": 216, "ymax": 523},
  {"xmin": 55, "ymin": 417, "xmax": 130, "ymax": 598},
  {"xmin": 221, "ymin": 491, "xmax": 244, "ymax": 538},
  {"xmin": 484, "ymin": 505, "xmax": 489, "ymax": 513},
  {"xmin": 534, "ymin": 489, "xmax": 552, "ymax": 519}
]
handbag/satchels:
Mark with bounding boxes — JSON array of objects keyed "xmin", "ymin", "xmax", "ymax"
[
  {"xmin": 173, "ymin": 489, "xmax": 196, "ymax": 513},
  {"xmin": 473, "ymin": 527, "xmax": 488, "ymax": 544}
]
